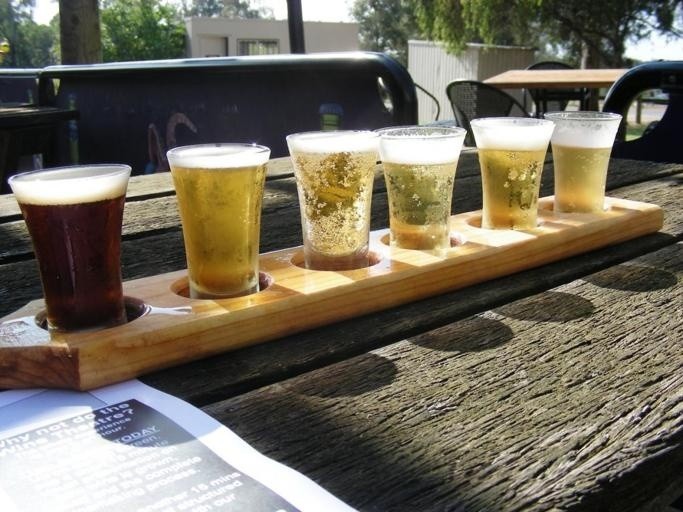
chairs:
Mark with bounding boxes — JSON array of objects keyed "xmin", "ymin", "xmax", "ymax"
[
  {"xmin": 602, "ymin": 62, "xmax": 683, "ymax": 164},
  {"xmin": 446, "ymin": 81, "xmax": 533, "ymax": 147},
  {"xmin": 523, "ymin": 61, "xmax": 585, "ymax": 117}
]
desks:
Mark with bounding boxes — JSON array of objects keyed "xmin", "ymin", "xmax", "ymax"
[
  {"xmin": 482, "ymin": 68, "xmax": 643, "ymax": 123},
  {"xmin": 0, "ymin": 107, "xmax": 80, "ymax": 194},
  {"xmin": 0, "ymin": 146, "xmax": 683, "ymax": 512}
]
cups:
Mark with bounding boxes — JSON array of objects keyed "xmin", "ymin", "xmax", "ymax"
[
  {"xmin": 542, "ymin": 111, "xmax": 622, "ymax": 212},
  {"xmin": 286, "ymin": 131, "xmax": 380, "ymax": 271},
  {"xmin": 375, "ymin": 125, "xmax": 468, "ymax": 249},
  {"xmin": 8, "ymin": 163, "xmax": 131, "ymax": 333},
  {"xmin": 166, "ymin": 143, "xmax": 271, "ymax": 299},
  {"xmin": 470, "ymin": 116, "xmax": 556, "ymax": 231}
]
